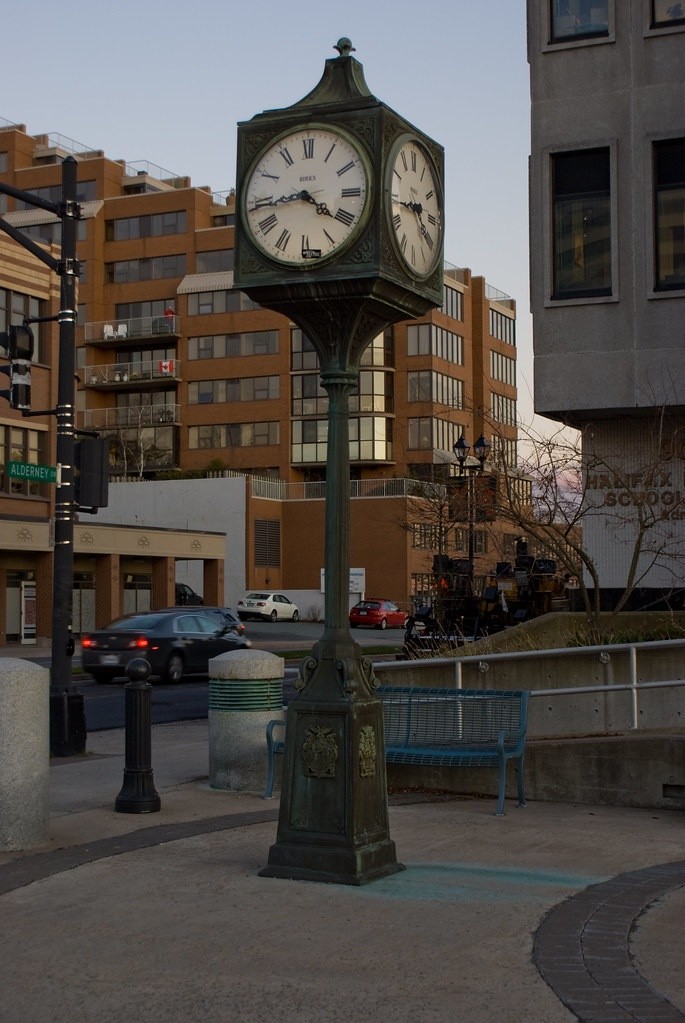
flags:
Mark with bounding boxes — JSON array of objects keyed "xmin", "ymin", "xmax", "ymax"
[{"xmin": 160, "ymin": 361, "xmax": 173, "ymax": 373}]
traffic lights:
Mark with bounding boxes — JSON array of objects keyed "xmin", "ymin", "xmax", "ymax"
[
  {"xmin": 74, "ymin": 436, "xmax": 109, "ymax": 507},
  {"xmin": 0, "ymin": 325, "xmax": 34, "ymax": 409}
]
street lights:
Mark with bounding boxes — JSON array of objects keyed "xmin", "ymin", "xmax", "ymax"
[{"xmin": 451, "ymin": 433, "xmax": 491, "ymax": 584}]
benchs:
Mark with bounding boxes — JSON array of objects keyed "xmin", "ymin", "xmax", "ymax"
[{"xmin": 265, "ymin": 686, "xmax": 532, "ymax": 817}]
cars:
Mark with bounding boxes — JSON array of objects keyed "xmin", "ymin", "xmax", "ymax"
[
  {"xmin": 81, "ymin": 605, "xmax": 254, "ymax": 684},
  {"xmin": 175, "ymin": 582, "xmax": 204, "ymax": 606},
  {"xmin": 236, "ymin": 592, "xmax": 301, "ymax": 622},
  {"xmin": 348, "ymin": 598, "xmax": 410, "ymax": 630}
]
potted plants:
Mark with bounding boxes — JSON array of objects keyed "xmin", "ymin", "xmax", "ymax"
[{"xmin": 225, "ymin": 187, "xmax": 236, "ymax": 206}]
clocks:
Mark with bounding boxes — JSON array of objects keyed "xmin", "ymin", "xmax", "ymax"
[
  {"xmin": 245, "ymin": 123, "xmax": 377, "ymax": 271},
  {"xmin": 383, "ymin": 132, "xmax": 442, "ymax": 283}
]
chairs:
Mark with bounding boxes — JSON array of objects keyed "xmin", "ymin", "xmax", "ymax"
[
  {"xmin": 115, "ymin": 324, "xmax": 128, "ymax": 338},
  {"xmin": 104, "ymin": 325, "xmax": 115, "ymax": 339}
]
